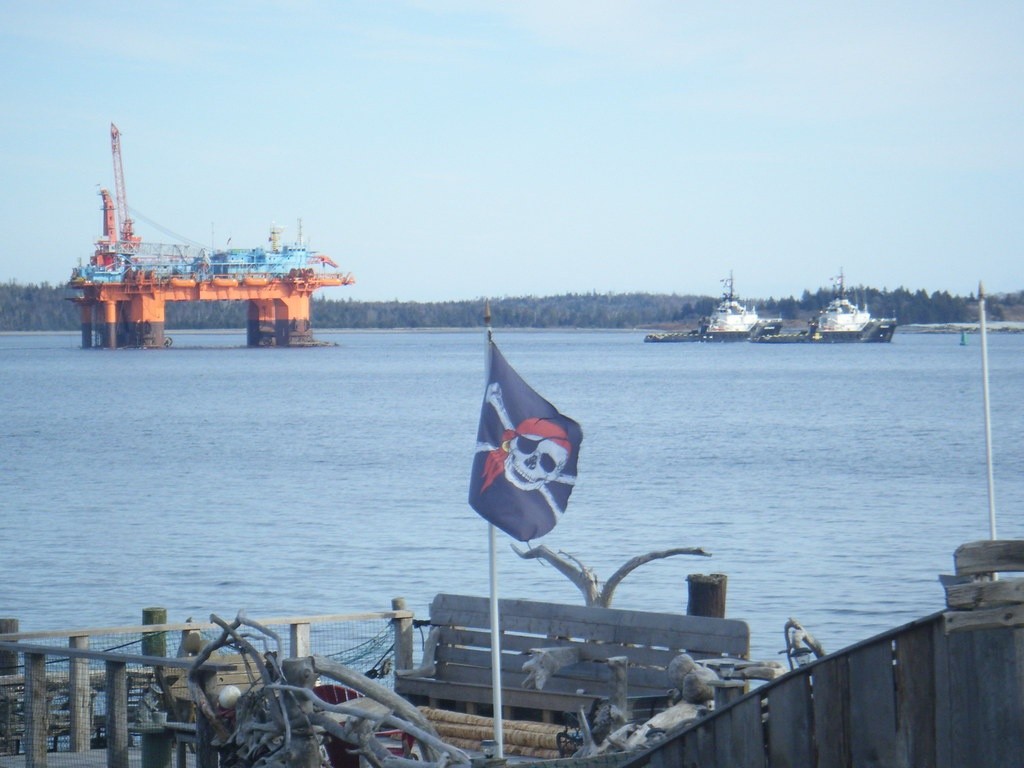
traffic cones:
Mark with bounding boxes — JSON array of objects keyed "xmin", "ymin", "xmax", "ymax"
[{"xmin": 959, "ymin": 329, "xmax": 969, "ymax": 345}]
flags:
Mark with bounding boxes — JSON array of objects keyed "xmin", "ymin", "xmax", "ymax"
[{"xmin": 468, "ymin": 340, "xmax": 582, "ymax": 544}]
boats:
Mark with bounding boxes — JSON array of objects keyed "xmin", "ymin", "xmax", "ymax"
[
  {"xmin": 745, "ymin": 268, "xmax": 896, "ymax": 345},
  {"xmin": 644, "ymin": 268, "xmax": 783, "ymax": 343}
]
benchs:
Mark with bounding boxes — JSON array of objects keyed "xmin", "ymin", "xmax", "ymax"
[
  {"xmin": 392, "ymin": 593, "xmax": 751, "ymax": 729},
  {"xmin": 555, "ymin": 692, "xmax": 670, "ymax": 760}
]
